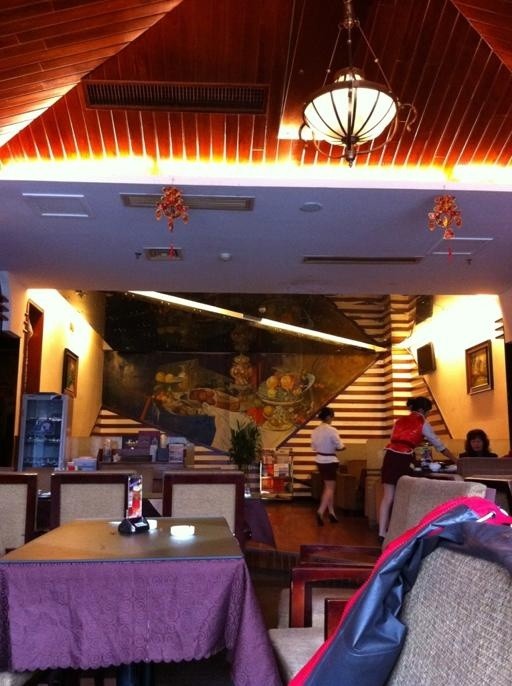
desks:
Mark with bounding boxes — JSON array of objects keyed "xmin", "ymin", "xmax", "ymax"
[{"xmin": 0, "ymin": 517, "xmax": 245, "ymax": 685}]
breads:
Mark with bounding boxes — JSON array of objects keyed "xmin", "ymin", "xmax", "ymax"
[{"xmin": 190, "ymin": 388, "xmax": 216, "ymax": 405}]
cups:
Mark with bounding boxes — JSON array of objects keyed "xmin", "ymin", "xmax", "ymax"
[
  {"xmin": 154, "ymin": 360, "xmax": 316, "ymax": 433},
  {"xmin": 67, "ymin": 462, "xmax": 76, "ymax": 471}
]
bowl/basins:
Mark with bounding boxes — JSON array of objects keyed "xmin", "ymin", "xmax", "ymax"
[{"xmin": 428, "ymin": 463, "xmax": 442, "ymax": 472}]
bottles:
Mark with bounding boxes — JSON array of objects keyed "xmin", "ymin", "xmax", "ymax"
[{"xmin": 184, "ymin": 443, "xmax": 195, "ymax": 468}]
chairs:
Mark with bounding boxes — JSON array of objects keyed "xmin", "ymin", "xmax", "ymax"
[
  {"xmin": 268, "ymin": 457, "xmax": 512, "ymax": 685},
  {"xmin": 0, "ymin": 455, "xmax": 245, "ymax": 557}
]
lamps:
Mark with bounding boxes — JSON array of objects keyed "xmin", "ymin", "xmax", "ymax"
[{"xmin": 299, "ymin": 0, "xmax": 418, "ymax": 165}]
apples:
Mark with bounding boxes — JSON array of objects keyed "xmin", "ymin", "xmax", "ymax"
[
  {"xmin": 154, "ymin": 371, "xmax": 174, "ymax": 384},
  {"xmin": 280, "ymin": 376, "xmax": 295, "ymax": 391},
  {"xmin": 266, "ymin": 374, "xmax": 278, "ymax": 387},
  {"xmin": 263, "ymin": 405, "xmax": 274, "ymax": 416},
  {"xmin": 247, "ymin": 393, "xmax": 264, "ymax": 407},
  {"xmin": 293, "ymin": 387, "xmax": 303, "ymax": 396},
  {"xmin": 267, "ymin": 390, "xmax": 278, "ymax": 399}
]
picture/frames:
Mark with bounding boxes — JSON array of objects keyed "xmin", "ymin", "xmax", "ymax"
[
  {"xmin": 417, "ymin": 342, "xmax": 436, "ymax": 376},
  {"xmin": 61, "ymin": 348, "xmax": 79, "ymax": 400},
  {"xmin": 465, "ymin": 339, "xmax": 494, "ymax": 396}
]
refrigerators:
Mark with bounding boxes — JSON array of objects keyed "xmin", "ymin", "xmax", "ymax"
[{"xmin": 19, "ymin": 394, "xmax": 75, "ymax": 499}]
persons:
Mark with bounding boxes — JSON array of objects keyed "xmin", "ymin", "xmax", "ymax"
[
  {"xmin": 311, "ymin": 407, "xmax": 346, "ymax": 527},
  {"xmin": 378, "ymin": 396, "xmax": 459, "ymax": 538},
  {"xmin": 459, "ymin": 429, "xmax": 498, "ymax": 458}
]
